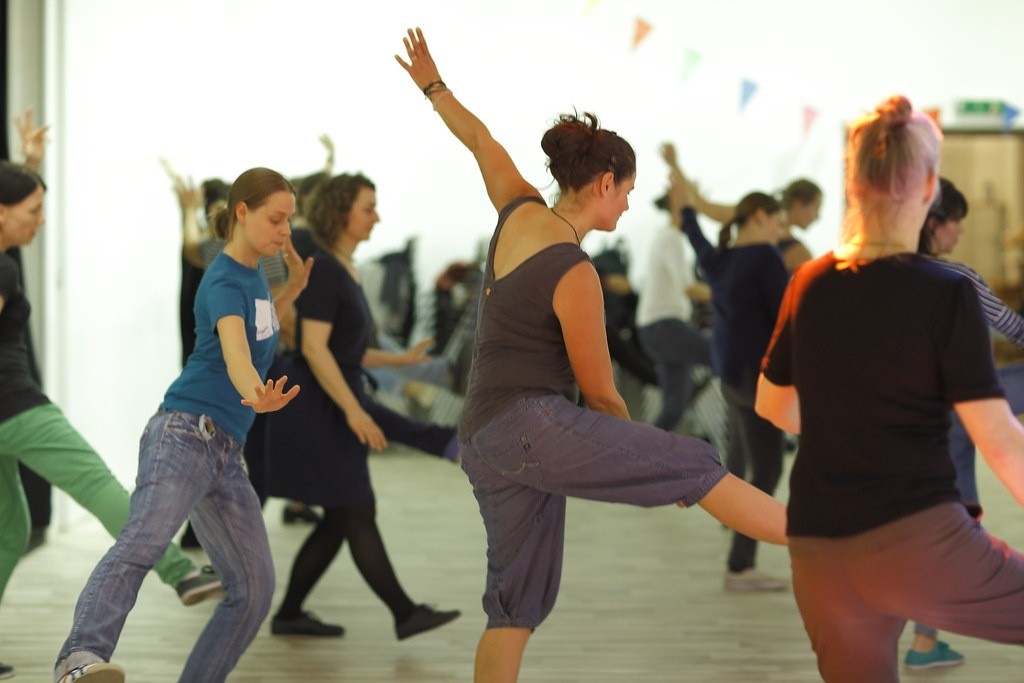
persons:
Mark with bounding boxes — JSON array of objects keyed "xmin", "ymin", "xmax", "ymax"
[
  {"xmin": 894, "ymin": 178, "xmax": 1023, "ymax": 667},
  {"xmin": 262, "ymin": 171, "xmax": 463, "ymax": 642},
  {"xmin": 172, "ymin": 135, "xmax": 822, "ymax": 487},
  {"xmin": 396, "ymin": 24, "xmax": 793, "ymax": 683},
  {"xmin": 1, "ymin": 111, "xmax": 238, "ymax": 678},
  {"xmin": 48, "ymin": 163, "xmax": 315, "ymax": 683},
  {"xmin": 659, "ymin": 177, "xmax": 801, "ymax": 590},
  {"xmin": 752, "ymin": 92, "xmax": 1024, "ymax": 682}
]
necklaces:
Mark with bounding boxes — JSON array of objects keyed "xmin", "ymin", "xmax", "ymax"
[{"xmin": 547, "ymin": 201, "xmax": 584, "ymax": 249}]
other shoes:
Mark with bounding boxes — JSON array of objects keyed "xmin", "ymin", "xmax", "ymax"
[
  {"xmin": 282, "ymin": 506, "xmax": 321, "ymax": 524},
  {"xmin": 905, "ymin": 640, "xmax": 964, "ymax": 669},
  {"xmin": 444, "ymin": 427, "xmax": 460, "ymax": 462},
  {"xmin": 396, "ymin": 603, "xmax": 461, "ymax": 640},
  {"xmin": 272, "ymin": 611, "xmax": 344, "ymax": 636},
  {"xmin": 724, "ymin": 567, "xmax": 787, "ymax": 593}
]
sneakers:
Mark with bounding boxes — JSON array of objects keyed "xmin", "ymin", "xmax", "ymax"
[
  {"xmin": 176, "ymin": 563, "xmax": 227, "ymax": 606},
  {"xmin": 58, "ymin": 661, "xmax": 126, "ymax": 683},
  {"xmin": 0, "ymin": 662, "xmax": 15, "ymax": 678}
]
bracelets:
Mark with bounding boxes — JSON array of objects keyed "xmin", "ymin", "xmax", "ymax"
[{"xmin": 423, "ymin": 80, "xmax": 452, "ymax": 112}]
